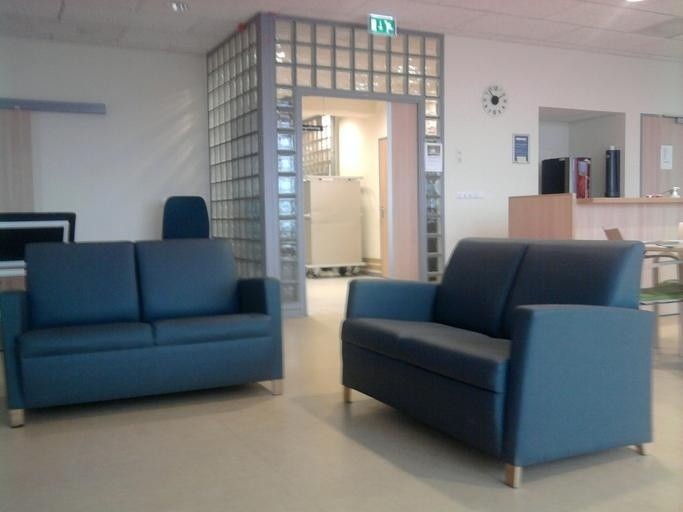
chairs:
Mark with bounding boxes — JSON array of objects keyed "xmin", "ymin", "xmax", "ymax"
[
  {"xmin": 162, "ymin": 196, "xmax": 209, "ymax": 236},
  {"xmin": 598, "ymin": 221, "xmax": 683, "ymax": 349}
]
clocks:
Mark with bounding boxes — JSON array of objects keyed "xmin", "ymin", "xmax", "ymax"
[{"xmin": 481, "ymin": 84, "xmax": 509, "ymax": 118}]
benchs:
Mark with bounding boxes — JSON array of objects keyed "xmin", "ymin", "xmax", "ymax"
[
  {"xmin": 0, "ymin": 236, "xmax": 284, "ymax": 429},
  {"xmin": 342, "ymin": 240, "xmax": 658, "ymax": 489}
]
